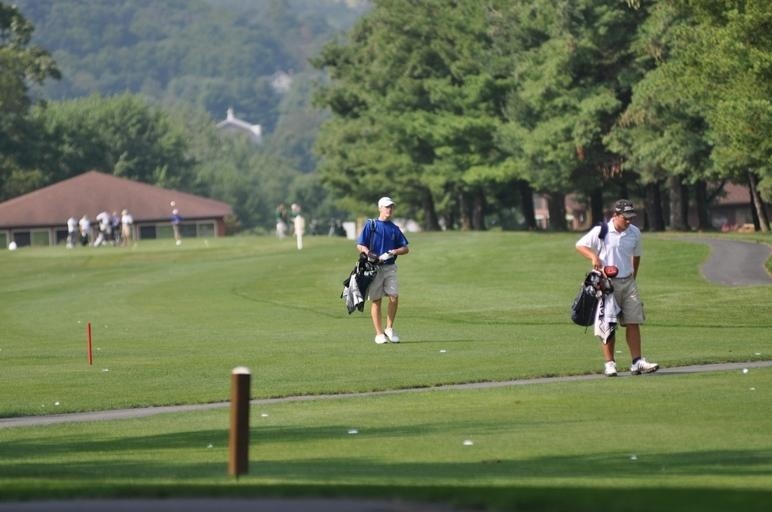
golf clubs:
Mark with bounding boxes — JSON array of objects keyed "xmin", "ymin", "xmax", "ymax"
[
  {"xmin": 584, "ymin": 273, "xmax": 614, "ymax": 300},
  {"xmin": 355, "ymin": 250, "xmax": 378, "ymax": 278}
]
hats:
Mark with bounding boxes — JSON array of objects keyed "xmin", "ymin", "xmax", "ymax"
[
  {"xmin": 377, "ymin": 196, "xmax": 396, "ymax": 208},
  {"xmin": 613, "ymin": 198, "xmax": 638, "ymax": 218}
]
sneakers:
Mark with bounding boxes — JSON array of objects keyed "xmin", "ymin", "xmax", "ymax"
[
  {"xmin": 385, "ymin": 327, "xmax": 400, "ymax": 343},
  {"xmin": 603, "ymin": 360, "xmax": 619, "ymax": 376},
  {"xmin": 375, "ymin": 333, "xmax": 388, "ymax": 344},
  {"xmin": 629, "ymin": 358, "xmax": 660, "ymax": 376}
]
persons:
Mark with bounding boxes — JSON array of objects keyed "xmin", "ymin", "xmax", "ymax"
[
  {"xmin": 289, "ymin": 213, "xmax": 306, "ymax": 249},
  {"xmin": 66, "ymin": 208, "xmax": 133, "ymax": 248},
  {"xmin": 169, "ymin": 209, "xmax": 182, "ymax": 245},
  {"xmin": 357, "ymin": 197, "xmax": 409, "ymax": 344},
  {"xmin": 575, "ymin": 198, "xmax": 659, "ymax": 377},
  {"xmin": 274, "ymin": 204, "xmax": 288, "ymax": 239}
]
